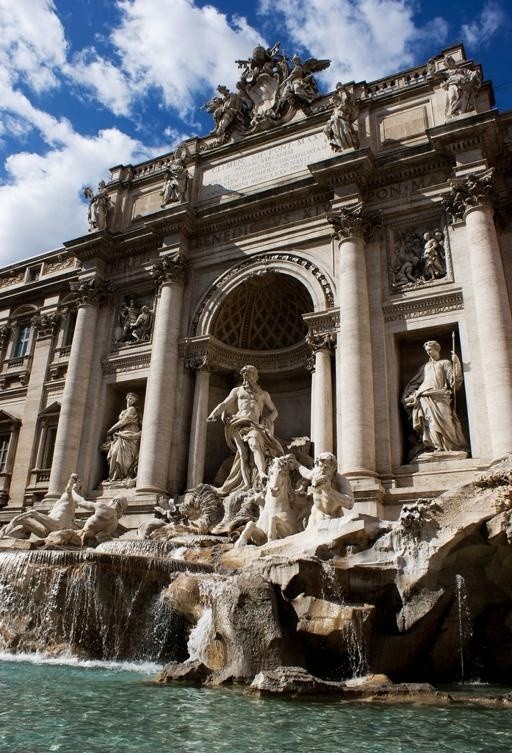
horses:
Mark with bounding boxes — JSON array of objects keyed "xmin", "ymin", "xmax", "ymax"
[{"xmin": 231, "ymin": 459, "xmax": 304, "ymax": 549}]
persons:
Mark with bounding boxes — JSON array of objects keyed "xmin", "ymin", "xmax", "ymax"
[
  {"xmin": 101, "ymin": 391, "xmax": 142, "ymax": 480},
  {"xmin": 156, "ymin": 366, "xmax": 353, "ymax": 546},
  {"xmin": 440, "ymin": 55, "xmax": 479, "ymax": 118},
  {"xmin": 86, "ymin": 181, "xmax": 111, "ymax": 232},
  {"xmin": 206, "ymin": 40, "xmax": 314, "ymax": 137},
  {"xmin": 46, "ymin": 482, "xmax": 128, "ymax": 547},
  {"xmin": 391, "ymin": 223, "xmax": 444, "ymax": 288},
  {"xmin": 325, "ymin": 81, "xmax": 360, "ymax": 151},
  {"xmin": 159, "ymin": 151, "xmax": 187, "ymax": 207},
  {"xmin": 117, "ymin": 298, "xmax": 152, "ymax": 343},
  {"xmin": 401, "ymin": 340, "xmax": 469, "ymax": 455}
]
